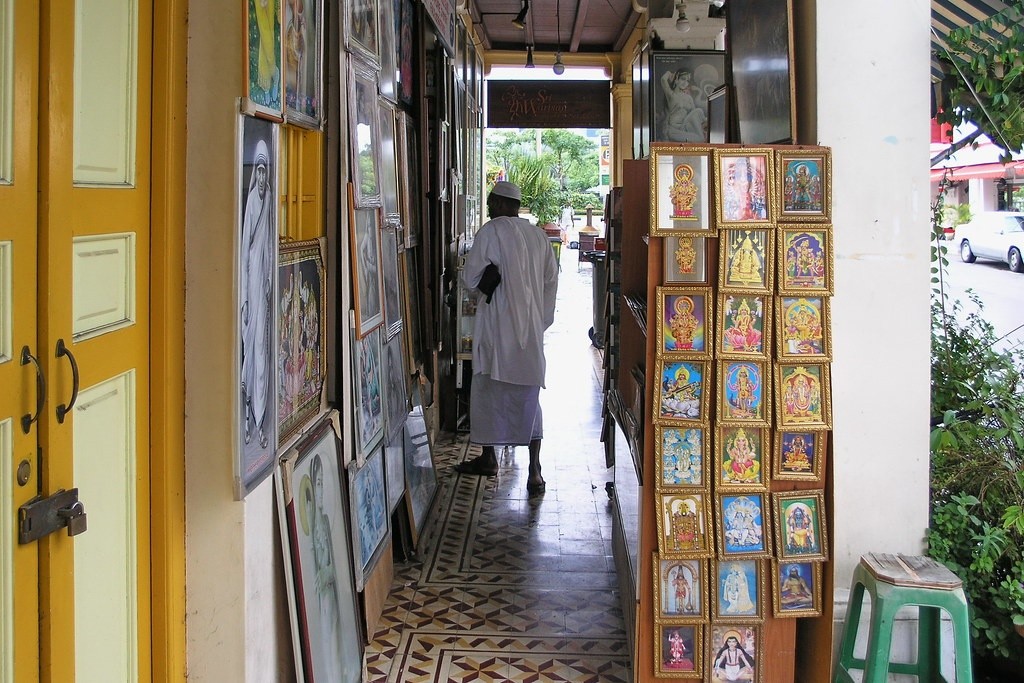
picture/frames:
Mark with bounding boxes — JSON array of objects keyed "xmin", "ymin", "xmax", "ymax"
[
  {"xmin": 231, "ymin": 0, "xmax": 481, "ymax": 683},
  {"xmin": 648, "ymin": 0, "xmax": 834, "ymax": 683}
]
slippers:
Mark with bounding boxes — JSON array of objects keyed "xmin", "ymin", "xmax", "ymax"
[
  {"xmin": 527, "ymin": 479, "xmax": 546, "ymax": 494},
  {"xmin": 453, "ymin": 458, "xmax": 498, "ymax": 476}
]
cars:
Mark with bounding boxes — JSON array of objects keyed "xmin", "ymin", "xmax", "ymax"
[{"xmin": 954, "ymin": 212, "xmax": 1024, "ymax": 271}]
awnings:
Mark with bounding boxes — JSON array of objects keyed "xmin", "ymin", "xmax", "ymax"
[{"xmin": 931, "ymin": 0, "xmax": 1024, "ymax": 121}]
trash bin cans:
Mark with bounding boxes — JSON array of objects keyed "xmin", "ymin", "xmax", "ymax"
[
  {"xmin": 548, "ymin": 236, "xmax": 562, "ymax": 274},
  {"xmin": 579, "ymin": 225, "xmax": 600, "ymax": 262}
]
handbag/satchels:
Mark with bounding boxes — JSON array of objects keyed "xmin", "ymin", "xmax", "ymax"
[{"xmin": 477, "ymin": 261, "xmax": 501, "ymax": 304}]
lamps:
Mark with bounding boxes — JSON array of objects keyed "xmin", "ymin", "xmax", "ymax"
[
  {"xmin": 525, "ymin": 45, "xmax": 536, "ymax": 68},
  {"xmin": 511, "ymin": 0, "xmax": 529, "ymax": 30}
]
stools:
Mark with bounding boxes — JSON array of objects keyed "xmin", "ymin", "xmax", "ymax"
[{"xmin": 836, "ymin": 553, "xmax": 973, "ymax": 683}]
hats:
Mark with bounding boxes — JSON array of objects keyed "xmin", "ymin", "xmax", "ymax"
[{"xmin": 491, "ymin": 181, "xmax": 522, "ymax": 201}]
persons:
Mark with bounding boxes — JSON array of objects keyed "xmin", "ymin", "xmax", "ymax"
[
  {"xmin": 558, "ymin": 200, "xmax": 574, "ymax": 244},
  {"xmin": 453, "ymin": 181, "xmax": 559, "ymax": 495}
]
